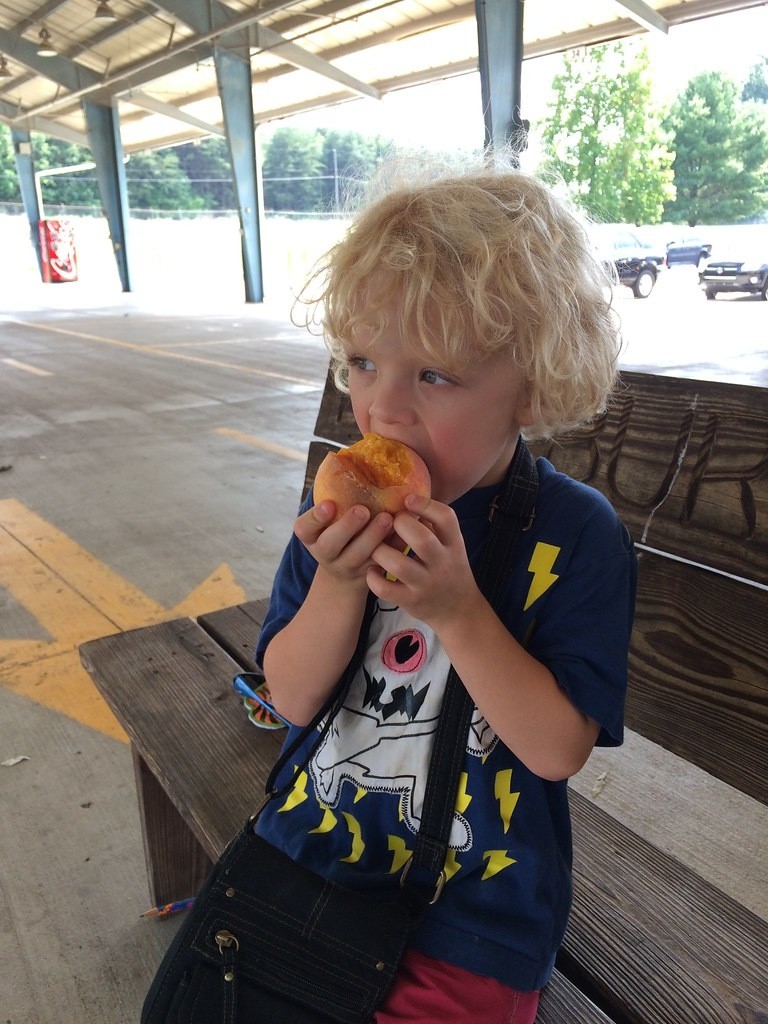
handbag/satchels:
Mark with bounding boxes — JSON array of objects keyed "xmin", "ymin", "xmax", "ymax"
[{"xmin": 139, "ymin": 828, "xmax": 406, "ymax": 1024}]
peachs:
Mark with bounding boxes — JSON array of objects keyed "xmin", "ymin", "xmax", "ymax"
[{"xmin": 314, "ymin": 431, "xmax": 432, "ymax": 539}]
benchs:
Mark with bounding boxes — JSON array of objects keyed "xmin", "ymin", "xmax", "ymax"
[{"xmin": 77, "ymin": 345, "xmax": 768, "ymax": 1024}]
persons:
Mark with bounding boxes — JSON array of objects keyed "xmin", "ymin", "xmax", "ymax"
[{"xmin": 254, "ymin": 174, "xmax": 637, "ymax": 1023}]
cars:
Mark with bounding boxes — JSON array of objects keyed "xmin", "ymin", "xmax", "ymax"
[
  {"xmin": 652, "ymin": 227, "xmax": 711, "ymax": 268},
  {"xmin": 699, "ymin": 238, "xmax": 768, "ymax": 300},
  {"xmin": 595, "ymin": 225, "xmax": 665, "ymax": 298}
]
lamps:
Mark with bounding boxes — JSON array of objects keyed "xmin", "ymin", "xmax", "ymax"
[
  {"xmin": 0, "ymin": 52, "xmax": 14, "ymax": 81},
  {"xmin": 36, "ymin": 27, "xmax": 57, "ymax": 57},
  {"xmin": 94, "ymin": 0, "xmax": 116, "ymax": 21}
]
